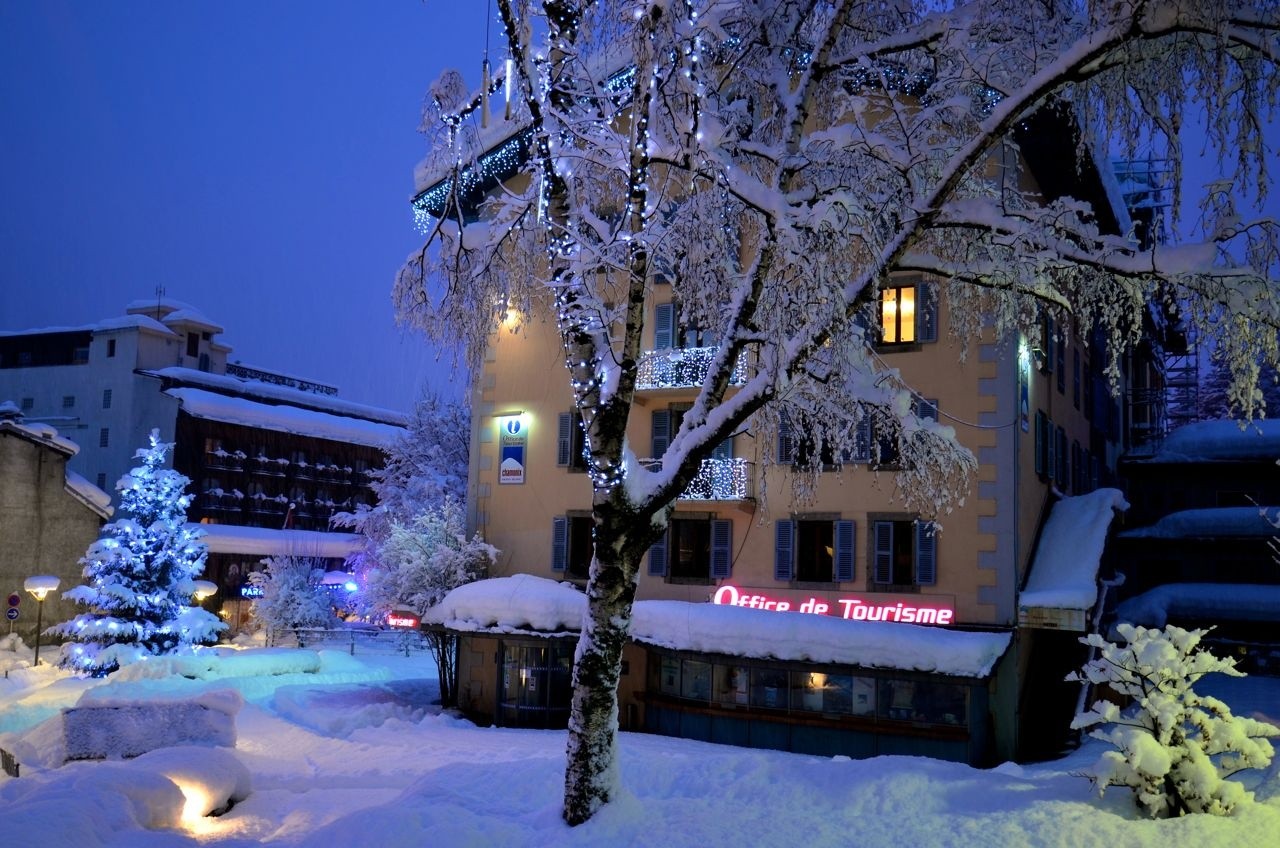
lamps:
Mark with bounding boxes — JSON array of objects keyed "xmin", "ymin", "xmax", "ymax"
[
  {"xmin": 191, "ymin": 581, "xmax": 219, "ymax": 600},
  {"xmin": 23, "ymin": 575, "xmax": 61, "ymax": 602},
  {"xmin": 738, "ymin": 501, "xmax": 756, "ymax": 515},
  {"xmin": 492, "ymin": 410, "xmax": 524, "ymax": 417}
]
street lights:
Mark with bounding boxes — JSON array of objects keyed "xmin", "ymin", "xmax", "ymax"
[
  {"xmin": 192, "ymin": 580, "xmax": 219, "ymax": 607},
  {"xmin": 24, "ymin": 575, "xmax": 61, "ymax": 667}
]
